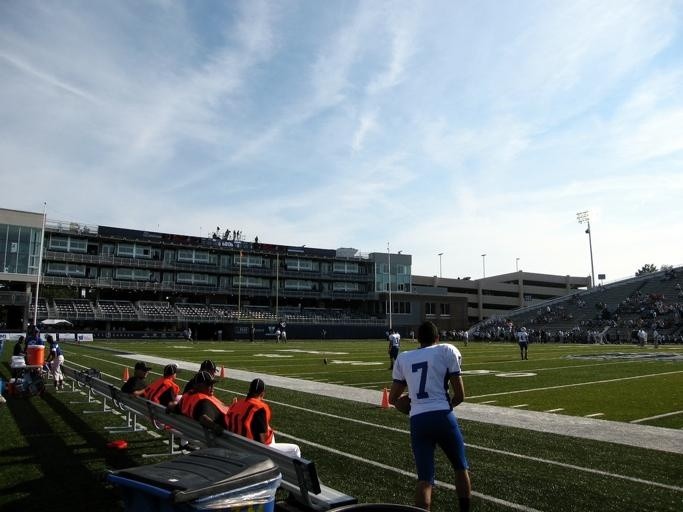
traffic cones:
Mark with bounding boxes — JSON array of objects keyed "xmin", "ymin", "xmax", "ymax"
[
  {"xmin": 121, "ymin": 366, "xmax": 130, "ymax": 383},
  {"xmin": 231, "ymin": 396, "xmax": 237, "ymax": 403},
  {"xmin": 377, "ymin": 386, "xmax": 394, "ymax": 408},
  {"xmin": 218, "ymin": 364, "xmax": 225, "ymax": 378}
]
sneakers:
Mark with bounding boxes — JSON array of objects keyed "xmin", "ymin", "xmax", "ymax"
[{"xmin": 520, "ymin": 356, "xmax": 529, "ymax": 361}]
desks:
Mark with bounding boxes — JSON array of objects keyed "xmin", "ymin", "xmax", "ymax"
[{"xmin": 9, "ymin": 365, "xmax": 43, "ymax": 399}]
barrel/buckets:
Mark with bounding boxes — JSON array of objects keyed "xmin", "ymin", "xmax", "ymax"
[{"xmin": 26, "ymin": 345, "xmax": 46, "ymax": 364}]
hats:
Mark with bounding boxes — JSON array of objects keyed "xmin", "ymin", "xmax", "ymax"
[
  {"xmin": 134, "ymin": 361, "xmax": 152, "ymax": 371},
  {"xmin": 200, "ymin": 359, "xmax": 220, "ymax": 372},
  {"xmin": 163, "ymin": 363, "xmax": 181, "ymax": 376},
  {"xmin": 194, "ymin": 371, "xmax": 220, "ymax": 385},
  {"xmin": 248, "ymin": 378, "xmax": 266, "ymax": 392}
]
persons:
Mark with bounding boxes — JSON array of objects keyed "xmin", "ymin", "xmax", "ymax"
[
  {"xmin": 182, "ymin": 360, "xmax": 217, "ymax": 395},
  {"xmin": 144, "ymin": 363, "xmax": 182, "ymax": 407},
  {"xmin": 27, "ymin": 321, "xmax": 41, "ymax": 344},
  {"xmin": 387, "ymin": 328, "xmax": 401, "ymax": 370},
  {"xmin": 408, "ymin": 267, "xmax": 682, "ymax": 348},
  {"xmin": 164, "ymin": 371, "xmax": 229, "ymax": 434},
  {"xmin": 281, "ymin": 328, "xmax": 288, "ymax": 344},
  {"xmin": 185, "ymin": 327, "xmax": 192, "ymax": 344},
  {"xmin": 320, "ymin": 327, "xmax": 327, "ymax": 343},
  {"xmin": 274, "ymin": 327, "xmax": 280, "ymax": 343},
  {"xmin": 386, "ymin": 319, "xmax": 474, "ymax": 511},
  {"xmin": 120, "ymin": 360, "xmax": 150, "ymax": 398},
  {"xmin": 217, "ymin": 328, "xmax": 223, "ymax": 342},
  {"xmin": 225, "ymin": 378, "xmax": 302, "ymax": 458},
  {"xmin": 516, "ymin": 326, "xmax": 529, "ymax": 361},
  {"xmin": 45, "ymin": 334, "xmax": 65, "ymax": 393},
  {"xmin": 12, "ymin": 335, "xmax": 25, "ymax": 357}
]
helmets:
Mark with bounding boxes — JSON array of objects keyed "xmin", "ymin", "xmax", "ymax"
[{"xmin": 520, "ymin": 326, "xmax": 527, "ymax": 332}]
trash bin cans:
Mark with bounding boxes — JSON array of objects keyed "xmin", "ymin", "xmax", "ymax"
[{"xmin": 107, "ymin": 446, "xmax": 283, "ymax": 511}]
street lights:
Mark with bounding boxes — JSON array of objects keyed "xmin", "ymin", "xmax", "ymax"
[
  {"xmin": 515, "ymin": 258, "xmax": 520, "ymax": 271},
  {"xmin": 576, "ymin": 209, "xmax": 594, "ymax": 288},
  {"xmin": 437, "ymin": 252, "xmax": 443, "ymax": 278},
  {"xmin": 481, "ymin": 254, "xmax": 486, "ymax": 279}
]
